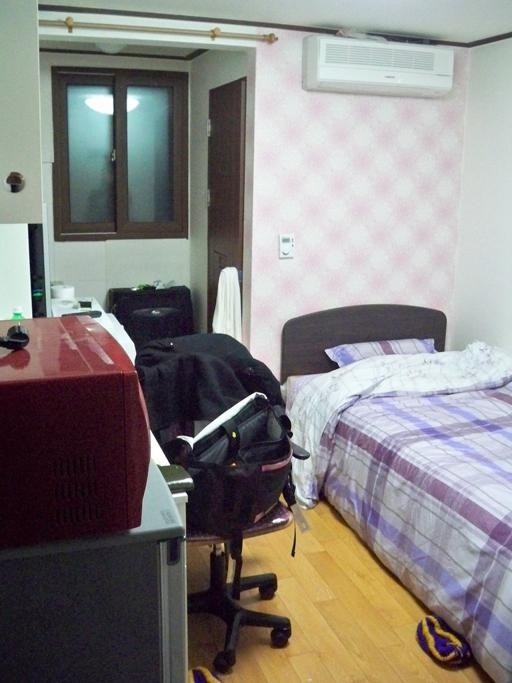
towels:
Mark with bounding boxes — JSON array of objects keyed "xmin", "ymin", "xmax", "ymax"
[{"xmin": 210, "ymin": 265, "xmax": 247, "ymax": 348}]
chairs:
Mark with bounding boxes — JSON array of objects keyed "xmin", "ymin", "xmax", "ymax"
[{"xmin": 178, "ymin": 432, "xmax": 297, "ymax": 674}]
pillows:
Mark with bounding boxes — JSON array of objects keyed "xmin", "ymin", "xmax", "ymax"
[{"xmin": 323, "ymin": 336, "xmax": 441, "ymax": 369}]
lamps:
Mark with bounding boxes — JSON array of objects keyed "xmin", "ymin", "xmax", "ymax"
[{"xmin": 84, "ymin": 93, "xmax": 141, "ymax": 116}]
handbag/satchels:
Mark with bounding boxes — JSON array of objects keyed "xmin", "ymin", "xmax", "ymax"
[{"xmin": 160, "ymin": 392, "xmax": 294, "ymax": 540}]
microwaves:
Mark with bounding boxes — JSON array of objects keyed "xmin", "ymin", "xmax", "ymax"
[{"xmin": 2, "ymin": 315, "xmax": 152, "ymax": 546}]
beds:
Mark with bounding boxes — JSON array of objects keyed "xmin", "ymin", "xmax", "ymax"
[{"xmin": 277, "ymin": 300, "xmax": 512, "ymax": 683}]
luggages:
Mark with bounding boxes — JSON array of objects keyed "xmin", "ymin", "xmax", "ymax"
[{"xmin": 108, "ymin": 285, "xmax": 193, "ymax": 352}]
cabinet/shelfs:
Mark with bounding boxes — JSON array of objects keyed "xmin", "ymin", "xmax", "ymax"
[{"xmin": 1, "ymin": 457, "xmax": 192, "ymax": 682}]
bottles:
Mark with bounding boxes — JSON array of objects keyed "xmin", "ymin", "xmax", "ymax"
[{"xmin": 9, "ymin": 308, "xmax": 26, "ymax": 320}]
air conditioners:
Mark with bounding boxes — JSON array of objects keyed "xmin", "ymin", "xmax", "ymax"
[{"xmin": 301, "ymin": 31, "xmax": 455, "ymax": 101}]
